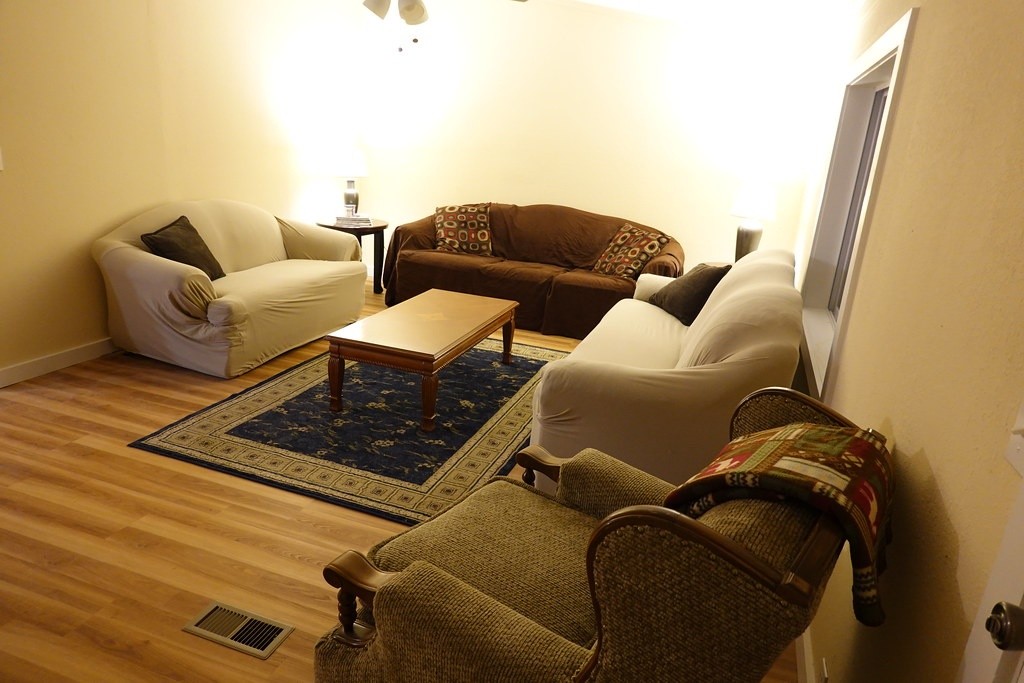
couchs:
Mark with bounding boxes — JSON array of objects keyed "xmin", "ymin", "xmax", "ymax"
[
  {"xmin": 529, "ymin": 249, "xmax": 804, "ymax": 495},
  {"xmin": 89, "ymin": 198, "xmax": 368, "ymax": 378},
  {"xmin": 383, "ymin": 203, "xmax": 685, "ymax": 340}
]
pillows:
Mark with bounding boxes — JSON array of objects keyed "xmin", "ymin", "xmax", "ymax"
[
  {"xmin": 649, "ymin": 263, "xmax": 732, "ymax": 326},
  {"xmin": 434, "ymin": 202, "xmax": 493, "ymax": 258},
  {"xmin": 140, "ymin": 215, "xmax": 226, "ymax": 281},
  {"xmin": 592, "ymin": 223, "xmax": 671, "ymax": 279}
]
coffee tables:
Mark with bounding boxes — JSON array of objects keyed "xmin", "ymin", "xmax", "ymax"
[{"xmin": 322, "ymin": 288, "xmax": 520, "ymax": 431}]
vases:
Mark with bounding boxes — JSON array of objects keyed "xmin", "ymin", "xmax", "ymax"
[{"xmin": 344, "ymin": 181, "xmax": 359, "ymax": 213}]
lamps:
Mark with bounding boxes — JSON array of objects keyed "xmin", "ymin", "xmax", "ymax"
[{"xmin": 363, "ymin": 0, "xmax": 428, "ymax": 52}]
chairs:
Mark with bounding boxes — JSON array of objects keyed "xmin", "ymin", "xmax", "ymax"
[{"xmin": 313, "ymin": 386, "xmax": 888, "ymax": 683}]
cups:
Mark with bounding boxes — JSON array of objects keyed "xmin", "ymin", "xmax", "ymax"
[{"xmin": 345, "ymin": 205, "xmax": 356, "ymax": 216}]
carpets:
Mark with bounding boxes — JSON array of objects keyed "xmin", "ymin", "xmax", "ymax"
[{"xmin": 127, "ymin": 338, "xmax": 572, "ymax": 526}]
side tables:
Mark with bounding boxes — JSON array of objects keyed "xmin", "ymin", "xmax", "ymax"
[{"xmin": 316, "ymin": 219, "xmax": 388, "ymax": 294}]
